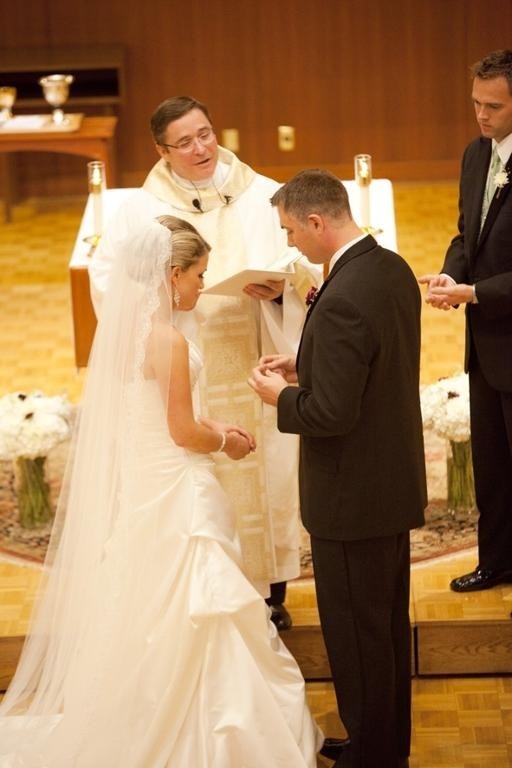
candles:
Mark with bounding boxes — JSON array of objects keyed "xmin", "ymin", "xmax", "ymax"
[
  {"xmin": 354, "ymin": 154, "xmax": 373, "ymax": 187},
  {"xmin": 87, "ymin": 160, "xmax": 107, "ymax": 192}
]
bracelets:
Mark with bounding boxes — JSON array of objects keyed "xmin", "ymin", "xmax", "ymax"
[{"xmin": 217, "ymin": 431, "xmax": 226, "ymax": 451}]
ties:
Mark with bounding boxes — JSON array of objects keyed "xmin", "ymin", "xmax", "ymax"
[{"xmin": 476, "ymin": 147, "xmax": 499, "ymax": 244}]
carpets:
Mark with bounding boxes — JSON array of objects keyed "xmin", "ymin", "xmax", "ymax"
[{"xmin": 1, "ymin": 454, "xmax": 481, "ymax": 590}]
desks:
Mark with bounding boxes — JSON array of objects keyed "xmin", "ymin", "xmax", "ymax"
[
  {"xmin": 65, "ymin": 177, "xmax": 400, "ymax": 375},
  {"xmin": 1, "ymin": 115, "xmax": 121, "ymax": 222}
]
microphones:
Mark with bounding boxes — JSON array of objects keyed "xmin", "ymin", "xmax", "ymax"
[{"xmin": 192, "ymin": 198, "xmax": 203, "ymax": 213}]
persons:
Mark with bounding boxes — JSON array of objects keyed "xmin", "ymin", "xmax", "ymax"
[
  {"xmin": 2, "ymin": 208, "xmax": 326, "ymax": 768},
  {"xmin": 415, "ymin": 47, "xmax": 512, "ymax": 592},
  {"xmin": 246, "ymin": 168, "xmax": 429, "ymax": 767},
  {"xmin": 133, "ymin": 94, "xmax": 327, "ymax": 634}
]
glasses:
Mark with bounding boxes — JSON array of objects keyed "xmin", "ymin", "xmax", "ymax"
[{"xmin": 158, "ymin": 128, "xmax": 216, "ymax": 153}]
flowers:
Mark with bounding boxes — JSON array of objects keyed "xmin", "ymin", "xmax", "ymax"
[
  {"xmin": 417, "ymin": 370, "xmax": 473, "ymax": 443},
  {"xmin": 0, "ymin": 385, "xmax": 78, "ymax": 460}
]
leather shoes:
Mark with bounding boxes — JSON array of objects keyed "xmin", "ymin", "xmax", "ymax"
[
  {"xmin": 269, "ymin": 604, "xmax": 292, "ymax": 631},
  {"xmin": 449, "ymin": 565, "xmax": 512, "ymax": 593},
  {"xmin": 320, "ymin": 735, "xmax": 351, "ymax": 761}
]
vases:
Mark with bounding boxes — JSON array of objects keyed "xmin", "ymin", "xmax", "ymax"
[
  {"xmin": 17, "ymin": 460, "xmax": 55, "ymax": 526},
  {"xmin": 445, "ymin": 436, "xmax": 478, "ymax": 521}
]
